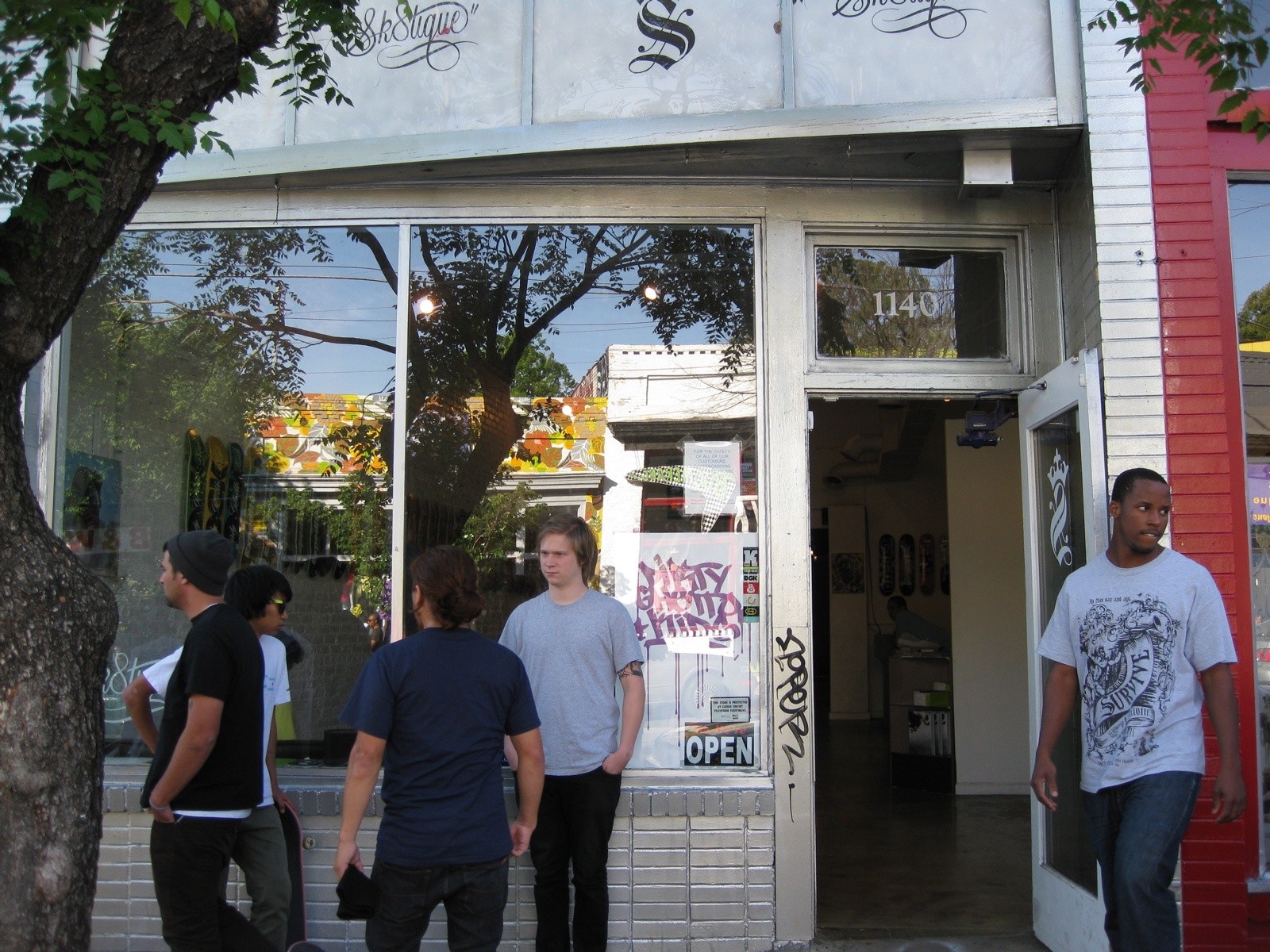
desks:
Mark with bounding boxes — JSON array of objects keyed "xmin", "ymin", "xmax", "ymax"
[{"xmin": 874, "ymin": 635, "xmax": 957, "ymax": 796}]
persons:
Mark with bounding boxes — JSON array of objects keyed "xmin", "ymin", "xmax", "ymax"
[
  {"xmin": 341, "ymin": 573, "xmax": 354, "ymax": 612},
  {"xmin": 367, "ymin": 612, "xmax": 384, "ymax": 653},
  {"xmin": 334, "ymin": 545, "xmax": 546, "ymax": 952},
  {"xmin": 1031, "ymin": 468, "xmax": 1246, "ymax": 952},
  {"xmin": 122, "ymin": 564, "xmax": 309, "ymax": 952},
  {"xmin": 140, "ymin": 530, "xmax": 270, "ymax": 952},
  {"xmin": 499, "ymin": 514, "xmax": 647, "ymax": 952}
]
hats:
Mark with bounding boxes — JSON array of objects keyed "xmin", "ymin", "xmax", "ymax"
[{"xmin": 168, "ymin": 530, "xmax": 237, "ymax": 597}]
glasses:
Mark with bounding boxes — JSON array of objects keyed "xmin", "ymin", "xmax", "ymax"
[{"xmin": 269, "ymin": 599, "xmax": 286, "ymax": 614}]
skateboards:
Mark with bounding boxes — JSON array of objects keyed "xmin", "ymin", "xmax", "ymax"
[
  {"xmin": 272, "ymin": 799, "xmax": 315, "ymax": 950},
  {"xmin": 287, "ymin": 941, "xmax": 326, "ymax": 952},
  {"xmin": 898, "ymin": 533, "xmax": 915, "ymax": 595},
  {"xmin": 185, "ymin": 428, "xmax": 456, "ymax": 579},
  {"xmin": 878, "ymin": 534, "xmax": 895, "ymax": 595},
  {"xmin": 939, "ymin": 534, "xmax": 950, "ymax": 596},
  {"xmin": 920, "ymin": 534, "xmax": 935, "ymax": 596}
]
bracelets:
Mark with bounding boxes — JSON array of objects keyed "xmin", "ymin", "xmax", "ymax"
[{"xmin": 149, "ymin": 798, "xmax": 166, "ymax": 811}]
